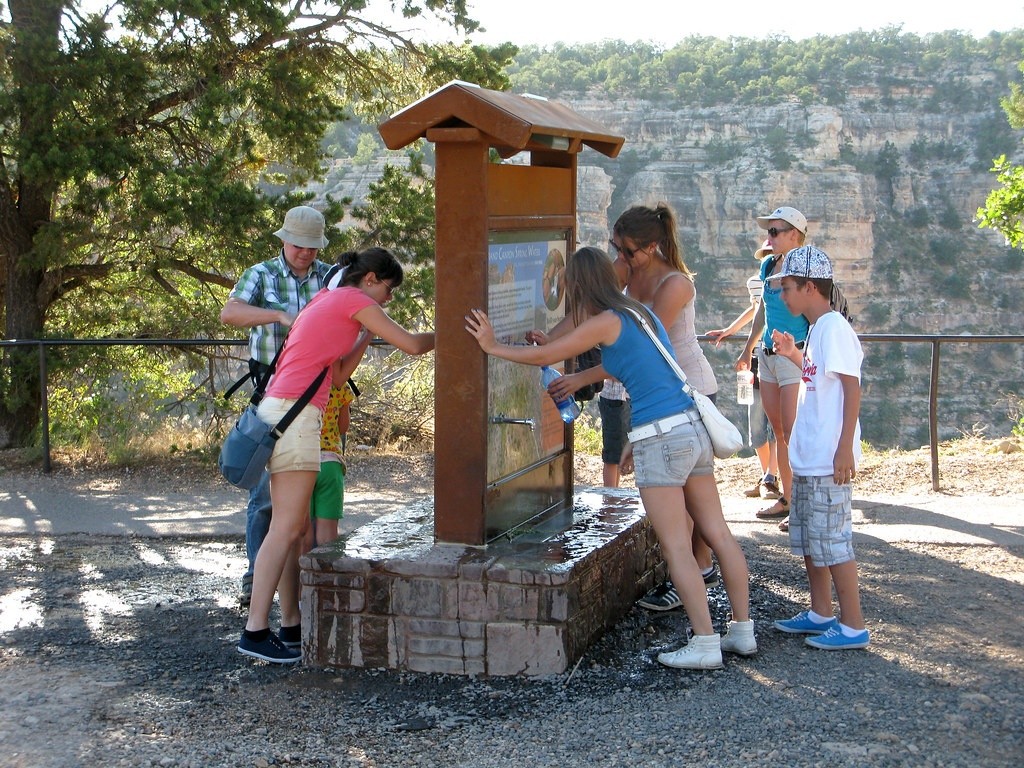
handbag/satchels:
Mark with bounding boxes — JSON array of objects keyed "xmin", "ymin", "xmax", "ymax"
[
  {"xmin": 218, "ymin": 406, "xmax": 280, "ymax": 490},
  {"xmin": 574, "ymin": 350, "xmax": 604, "ymax": 401},
  {"xmin": 691, "ymin": 390, "xmax": 743, "ymax": 460}
]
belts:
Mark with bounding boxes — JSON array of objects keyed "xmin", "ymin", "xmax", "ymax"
[
  {"xmin": 627, "ymin": 409, "xmax": 701, "ymax": 443},
  {"xmin": 764, "ymin": 342, "xmax": 804, "ymax": 357}
]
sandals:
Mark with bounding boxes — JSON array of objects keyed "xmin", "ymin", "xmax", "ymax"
[
  {"xmin": 756, "ymin": 497, "xmax": 790, "ymax": 518},
  {"xmin": 778, "ymin": 516, "xmax": 790, "ymax": 530}
]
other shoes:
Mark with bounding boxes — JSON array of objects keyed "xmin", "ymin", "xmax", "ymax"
[{"xmin": 238, "ymin": 576, "xmax": 253, "ymax": 603}]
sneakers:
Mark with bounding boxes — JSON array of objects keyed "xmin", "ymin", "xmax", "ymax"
[
  {"xmin": 720, "ymin": 613, "xmax": 758, "ymax": 656},
  {"xmin": 701, "ymin": 565, "xmax": 720, "ymax": 589},
  {"xmin": 743, "ymin": 476, "xmax": 779, "ymax": 499},
  {"xmin": 278, "ymin": 630, "xmax": 302, "ymax": 646},
  {"xmin": 804, "ymin": 623, "xmax": 869, "ymax": 650},
  {"xmin": 640, "ymin": 579, "xmax": 684, "ymax": 610},
  {"xmin": 237, "ymin": 630, "xmax": 302, "ymax": 662},
  {"xmin": 774, "ymin": 608, "xmax": 838, "ymax": 635},
  {"xmin": 657, "ymin": 627, "xmax": 722, "ymax": 670}
]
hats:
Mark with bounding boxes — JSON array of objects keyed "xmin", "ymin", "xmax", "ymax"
[
  {"xmin": 754, "ymin": 239, "xmax": 773, "ymax": 260},
  {"xmin": 756, "ymin": 207, "xmax": 808, "ymax": 237},
  {"xmin": 272, "ymin": 205, "xmax": 329, "ymax": 250},
  {"xmin": 765, "ymin": 245, "xmax": 833, "ymax": 283}
]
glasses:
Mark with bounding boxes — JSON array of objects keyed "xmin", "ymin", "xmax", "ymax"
[
  {"xmin": 609, "ymin": 238, "xmax": 652, "ymax": 258},
  {"xmin": 293, "ymin": 245, "xmax": 318, "ymax": 251},
  {"xmin": 381, "ymin": 280, "xmax": 399, "ymax": 295},
  {"xmin": 768, "ymin": 227, "xmax": 792, "ymax": 237}
]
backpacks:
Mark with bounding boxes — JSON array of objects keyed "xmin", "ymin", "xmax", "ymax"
[{"xmin": 765, "ymin": 255, "xmax": 854, "ymax": 323}]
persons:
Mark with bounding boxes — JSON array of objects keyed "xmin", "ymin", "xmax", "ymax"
[
  {"xmin": 238, "ymin": 248, "xmax": 435, "ymax": 662},
  {"xmin": 220, "ymin": 205, "xmax": 353, "ymax": 629},
  {"xmin": 706, "ymin": 206, "xmax": 853, "ymax": 531},
  {"xmin": 524, "ymin": 206, "xmax": 718, "ymax": 612},
  {"xmin": 766, "ymin": 248, "xmax": 870, "ymax": 650},
  {"xmin": 465, "ymin": 247, "xmax": 757, "ymax": 668}
]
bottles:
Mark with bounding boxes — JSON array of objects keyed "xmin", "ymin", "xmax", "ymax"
[
  {"xmin": 736, "ymin": 365, "xmax": 755, "ymax": 404},
  {"xmin": 539, "ymin": 364, "xmax": 580, "ymax": 425}
]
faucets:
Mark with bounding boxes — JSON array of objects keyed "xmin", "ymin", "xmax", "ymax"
[{"xmin": 489, "ymin": 410, "xmax": 537, "ymax": 432}]
